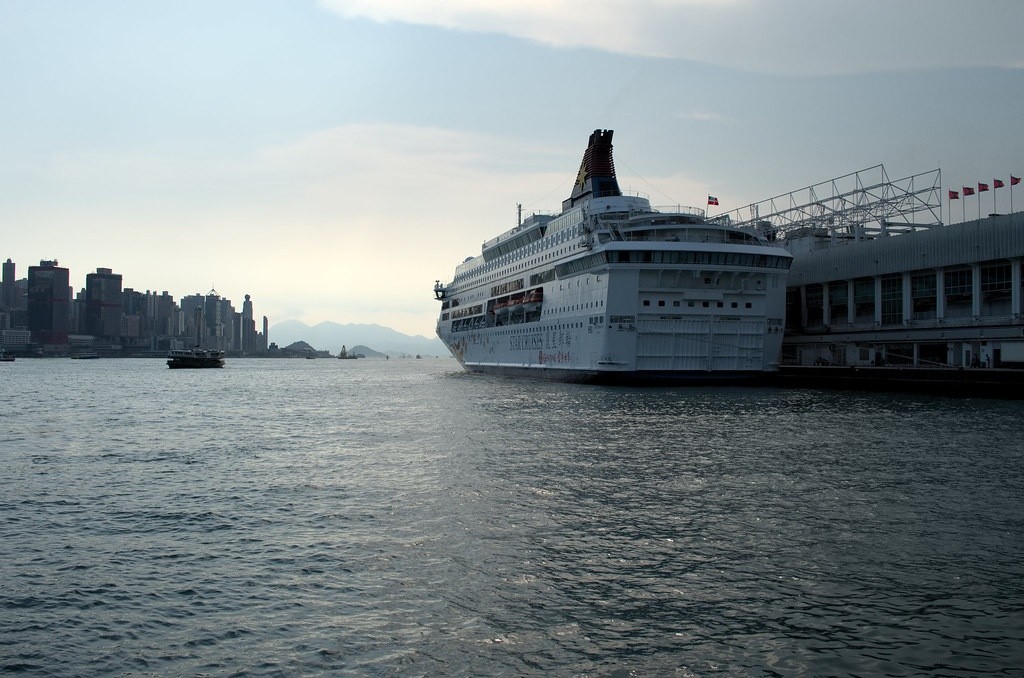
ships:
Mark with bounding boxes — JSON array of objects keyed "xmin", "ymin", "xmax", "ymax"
[{"xmin": 433, "ymin": 128, "xmax": 794, "ymax": 388}]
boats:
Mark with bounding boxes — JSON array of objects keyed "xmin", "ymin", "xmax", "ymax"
[
  {"xmin": 506, "ymin": 296, "xmax": 525, "ymax": 312},
  {"xmin": 71, "ymin": 351, "xmax": 102, "ymax": 359},
  {"xmin": 166, "ymin": 344, "xmax": 227, "ymax": 368},
  {"xmin": 338, "ymin": 345, "xmax": 358, "ymax": 359},
  {"xmin": 306, "ymin": 355, "xmax": 316, "ymax": 359},
  {"xmin": 521, "ymin": 289, "xmax": 544, "ymax": 312},
  {"xmin": 0, "ymin": 349, "xmax": 16, "ymax": 362},
  {"xmin": 492, "ymin": 302, "xmax": 509, "ymax": 316}
]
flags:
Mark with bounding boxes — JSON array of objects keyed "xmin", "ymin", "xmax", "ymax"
[
  {"xmin": 979, "ymin": 183, "xmax": 989, "ymax": 191},
  {"xmin": 1012, "ymin": 177, "xmax": 1022, "ymax": 185},
  {"xmin": 708, "ymin": 196, "xmax": 718, "ymax": 205},
  {"xmin": 994, "ymin": 180, "xmax": 1004, "ymax": 188},
  {"xmin": 963, "ymin": 188, "xmax": 975, "ymax": 195},
  {"xmin": 949, "ymin": 191, "xmax": 959, "ymax": 199}
]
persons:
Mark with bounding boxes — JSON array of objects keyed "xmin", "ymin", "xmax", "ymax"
[
  {"xmin": 674, "ymin": 237, "xmax": 679, "ymax": 242},
  {"xmin": 970, "ymin": 359, "xmax": 977, "ymax": 368},
  {"xmin": 986, "ymin": 354, "xmax": 990, "ymax": 368}
]
